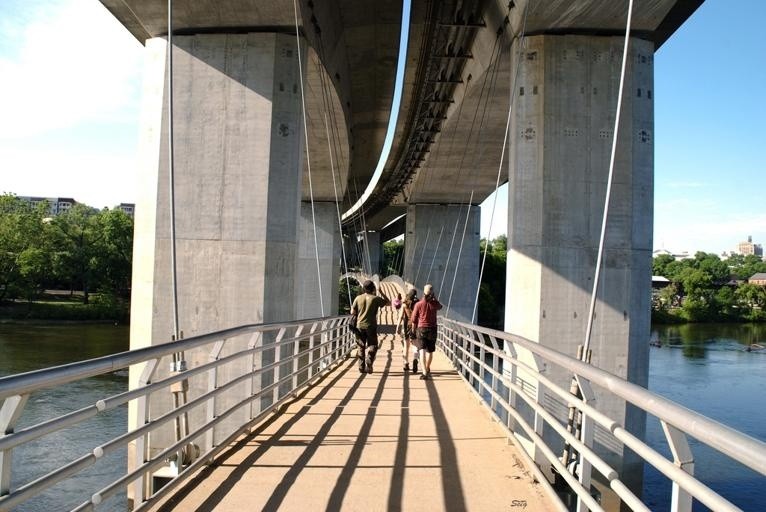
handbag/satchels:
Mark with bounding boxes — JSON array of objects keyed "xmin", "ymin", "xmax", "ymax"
[{"xmin": 348, "ymin": 314, "xmax": 356, "ymax": 334}]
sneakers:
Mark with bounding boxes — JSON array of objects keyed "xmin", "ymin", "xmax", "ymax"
[
  {"xmin": 358, "ymin": 363, "xmax": 372, "ymax": 374},
  {"xmin": 419, "ymin": 374, "xmax": 427, "ymax": 380},
  {"xmin": 412, "ymin": 359, "xmax": 417, "ymax": 373},
  {"xmin": 402, "ymin": 363, "xmax": 409, "ymax": 372}
]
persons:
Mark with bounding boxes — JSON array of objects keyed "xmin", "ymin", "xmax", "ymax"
[
  {"xmin": 411, "ymin": 284, "xmax": 443, "ymax": 379},
  {"xmin": 350, "ymin": 280, "xmax": 392, "ymax": 374},
  {"xmin": 396, "ymin": 289, "xmax": 420, "ymax": 372},
  {"xmin": 396, "ymin": 293, "xmax": 402, "ymax": 317}
]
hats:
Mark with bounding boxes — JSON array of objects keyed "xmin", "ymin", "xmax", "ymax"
[{"xmin": 423, "ymin": 283, "xmax": 432, "ymax": 295}]
